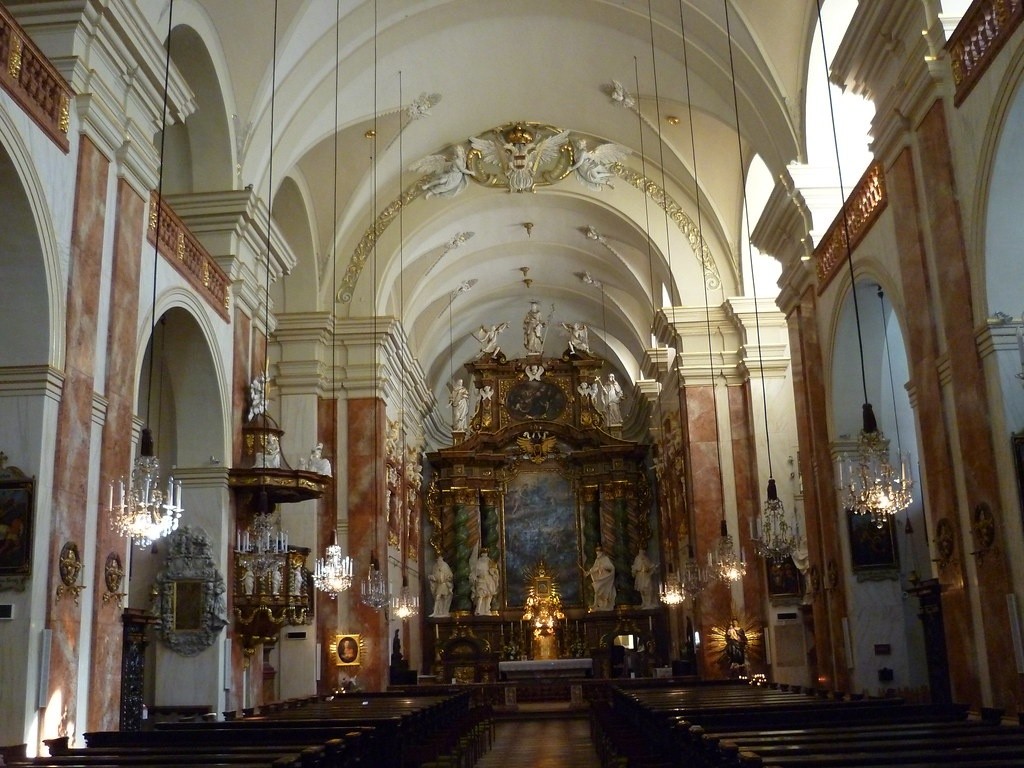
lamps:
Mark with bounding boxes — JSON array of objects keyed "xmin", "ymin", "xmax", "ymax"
[
  {"xmin": 360, "ymin": 0, "xmax": 394, "ymax": 616},
  {"xmin": 812, "ymin": 0, "xmax": 911, "ymax": 529},
  {"xmin": 235, "ymin": 0, "xmax": 296, "ymax": 577},
  {"xmin": 388, "ymin": 72, "xmax": 418, "ymax": 620},
  {"xmin": 313, "ymin": 0, "xmax": 354, "ymax": 599},
  {"xmin": 720, "ymin": 0, "xmax": 803, "ymax": 567},
  {"xmin": 107, "ymin": 0, "xmax": 185, "ymax": 548},
  {"xmin": 646, "ymin": 0, "xmax": 717, "ymax": 599},
  {"xmin": 678, "ymin": 0, "xmax": 751, "ymax": 582},
  {"xmin": 634, "ymin": 56, "xmax": 687, "ymax": 608}
]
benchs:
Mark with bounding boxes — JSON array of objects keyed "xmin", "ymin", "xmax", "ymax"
[
  {"xmin": 0, "ymin": 685, "xmax": 496, "ymax": 768},
  {"xmin": 589, "ymin": 678, "xmax": 1024, "ymax": 768}
]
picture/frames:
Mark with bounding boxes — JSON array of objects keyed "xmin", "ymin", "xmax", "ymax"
[
  {"xmin": 335, "ymin": 634, "xmax": 360, "ymax": 666},
  {"xmin": 534, "ymin": 577, "xmax": 552, "ymax": 597}
]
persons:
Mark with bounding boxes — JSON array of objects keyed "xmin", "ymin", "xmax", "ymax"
[
  {"xmin": 469, "ymin": 548, "xmax": 497, "ymax": 617},
  {"xmin": 428, "ymin": 556, "xmax": 454, "ymax": 618},
  {"xmin": 631, "ymin": 546, "xmax": 660, "ymax": 610},
  {"xmin": 584, "ymin": 547, "xmax": 615, "ymax": 612}
]
